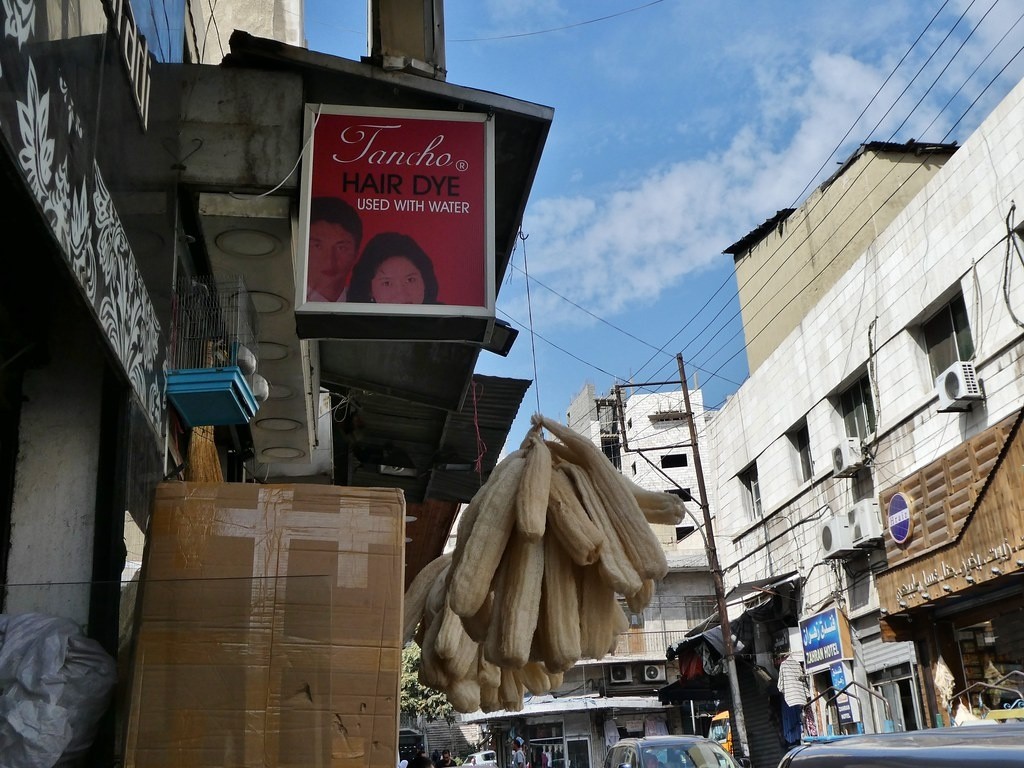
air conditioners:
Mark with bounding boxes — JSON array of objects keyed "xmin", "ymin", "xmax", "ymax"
[
  {"xmin": 644, "ymin": 664, "xmax": 667, "ymax": 682},
  {"xmin": 848, "ymin": 498, "xmax": 883, "ymax": 549},
  {"xmin": 609, "ymin": 665, "xmax": 634, "ymax": 684},
  {"xmin": 933, "ymin": 361, "xmax": 988, "ymax": 414},
  {"xmin": 819, "ymin": 514, "xmax": 854, "ymax": 560},
  {"xmin": 831, "ymin": 436, "xmax": 872, "ymax": 480}
]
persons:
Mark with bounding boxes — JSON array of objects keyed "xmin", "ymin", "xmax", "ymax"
[
  {"xmin": 346, "ymin": 230, "xmax": 446, "ymax": 305},
  {"xmin": 305, "ymin": 195, "xmax": 364, "ymax": 303},
  {"xmin": 512, "ymin": 740, "xmax": 526, "ymax": 768},
  {"xmin": 407, "ymin": 749, "xmax": 457, "ymax": 768}
]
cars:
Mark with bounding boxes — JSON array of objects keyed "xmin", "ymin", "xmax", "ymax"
[{"xmin": 461, "ymin": 750, "xmax": 497, "ymax": 768}]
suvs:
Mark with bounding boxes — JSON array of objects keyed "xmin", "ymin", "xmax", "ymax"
[{"xmin": 602, "ymin": 734, "xmax": 751, "ymax": 768}]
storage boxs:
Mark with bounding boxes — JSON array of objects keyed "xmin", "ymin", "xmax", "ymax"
[{"xmin": 127, "ymin": 476, "xmax": 407, "ymax": 767}]
